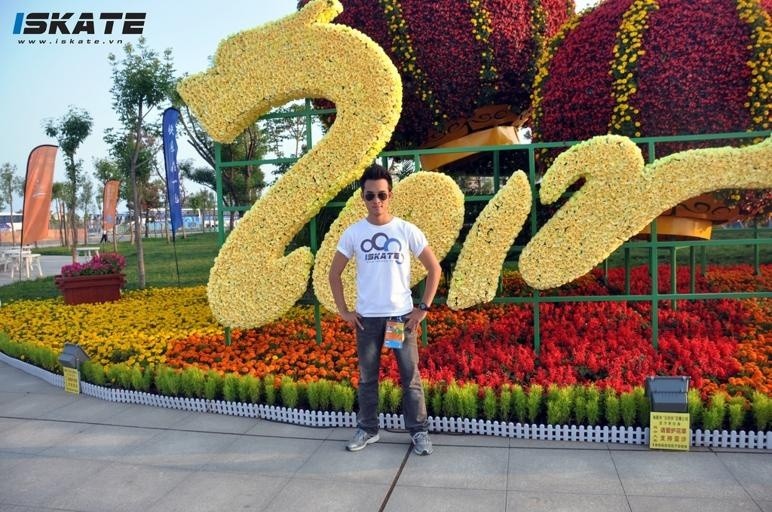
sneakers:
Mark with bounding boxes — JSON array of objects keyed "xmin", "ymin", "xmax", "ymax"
[
  {"xmin": 345, "ymin": 428, "xmax": 380, "ymax": 450},
  {"xmin": 410, "ymin": 430, "xmax": 434, "ymax": 455}
]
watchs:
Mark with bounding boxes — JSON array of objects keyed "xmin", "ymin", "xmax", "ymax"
[{"xmin": 419, "ymin": 302, "xmax": 430, "ymax": 311}]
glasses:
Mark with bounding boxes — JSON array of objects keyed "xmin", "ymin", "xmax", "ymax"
[{"xmin": 363, "ymin": 191, "xmax": 387, "ymax": 200}]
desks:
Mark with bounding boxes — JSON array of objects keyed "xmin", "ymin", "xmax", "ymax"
[
  {"xmin": 77, "ymin": 247, "xmax": 100, "ymax": 262},
  {"xmin": 0, "ymin": 246, "xmax": 42, "ymax": 279}
]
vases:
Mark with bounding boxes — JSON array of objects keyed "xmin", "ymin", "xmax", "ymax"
[{"xmin": 55, "ymin": 274, "xmax": 125, "ymax": 306}]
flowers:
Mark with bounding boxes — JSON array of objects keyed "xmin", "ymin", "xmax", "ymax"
[{"xmin": 60, "ymin": 253, "xmax": 125, "ymax": 276}]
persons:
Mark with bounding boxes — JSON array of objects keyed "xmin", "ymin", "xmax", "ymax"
[
  {"xmin": 328, "ymin": 163, "xmax": 442, "ymax": 454},
  {"xmin": 100, "ymin": 225, "xmax": 107, "ymax": 243}
]
